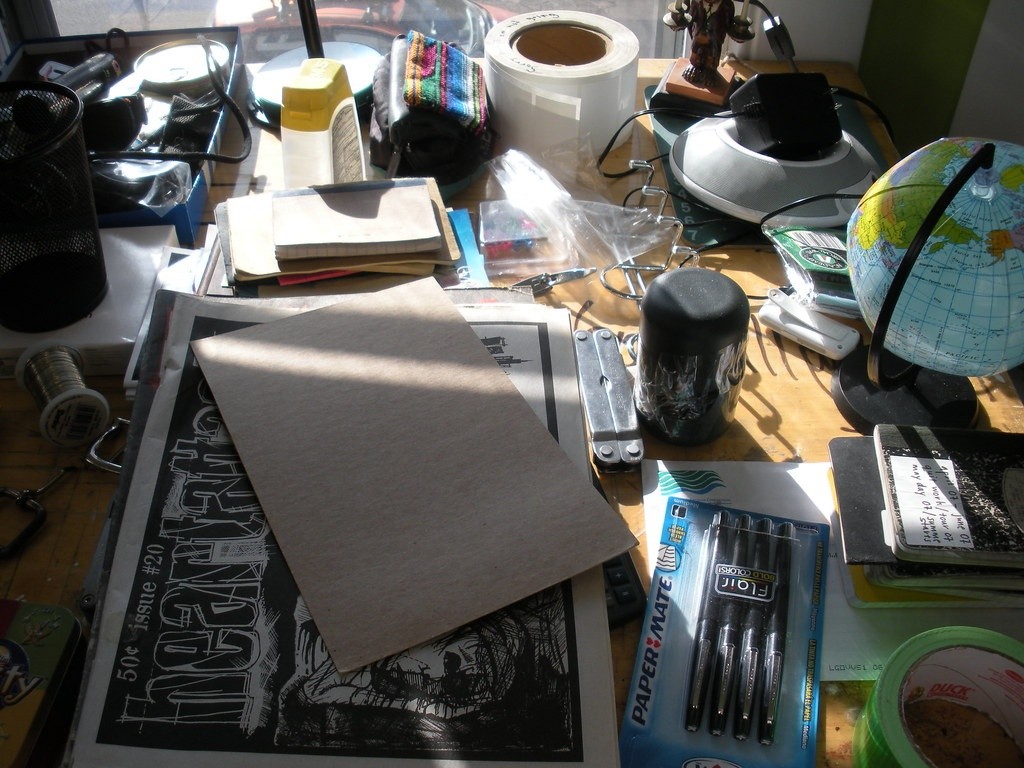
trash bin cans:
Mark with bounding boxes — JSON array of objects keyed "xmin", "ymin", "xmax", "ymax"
[{"xmin": 0, "ymin": 81, "xmax": 107, "ymax": 335}]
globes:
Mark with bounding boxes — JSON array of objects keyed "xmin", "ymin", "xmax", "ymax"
[{"xmin": 831, "ymin": 136, "xmax": 1024, "ymax": 436}]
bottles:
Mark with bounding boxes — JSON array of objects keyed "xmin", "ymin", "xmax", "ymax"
[
  {"xmin": 16, "ymin": 51, "xmax": 125, "ymax": 137},
  {"xmin": 281, "ymin": 56, "xmax": 367, "ymax": 196}
]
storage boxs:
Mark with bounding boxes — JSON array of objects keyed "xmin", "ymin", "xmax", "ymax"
[{"xmin": 0, "ymin": 24, "xmax": 244, "ymax": 244}]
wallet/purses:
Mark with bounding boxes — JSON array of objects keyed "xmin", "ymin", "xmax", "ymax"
[{"xmin": 369, "ymin": 27, "xmax": 491, "ymax": 181}]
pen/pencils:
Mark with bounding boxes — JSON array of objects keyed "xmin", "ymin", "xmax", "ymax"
[{"xmin": 685, "ymin": 510, "xmax": 794, "ymax": 747}]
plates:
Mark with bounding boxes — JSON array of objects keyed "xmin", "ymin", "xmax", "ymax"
[{"xmin": 249, "ymin": 41, "xmax": 383, "ymax": 126}]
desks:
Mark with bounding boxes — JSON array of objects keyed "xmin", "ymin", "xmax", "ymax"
[{"xmin": 0, "ymin": 60, "xmax": 1024, "ymax": 768}]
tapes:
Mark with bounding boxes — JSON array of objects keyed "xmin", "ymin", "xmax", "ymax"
[
  {"xmin": 483, "ymin": 9, "xmax": 640, "ymax": 161},
  {"xmin": 850, "ymin": 625, "xmax": 1024, "ymax": 768}
]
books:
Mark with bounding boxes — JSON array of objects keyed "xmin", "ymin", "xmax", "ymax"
[
  {"xmin": 830, "ymin": 421, "xmax": 1023, "ymax": 609},
  {"xmin": 195, "ymin": 172, "xmax": 492, "ymax": 295}
]
